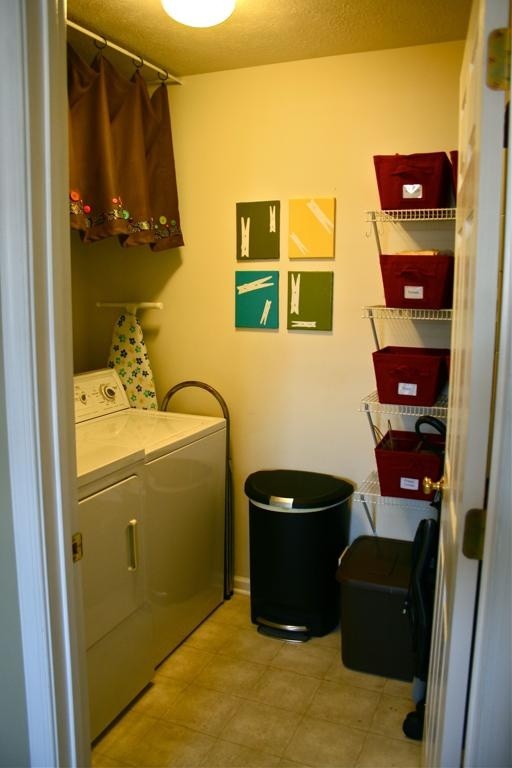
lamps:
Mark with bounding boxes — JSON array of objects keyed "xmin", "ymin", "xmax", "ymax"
[{"xmin": 158, "ymin": 0, "xmax": 243, "ymax": 27}]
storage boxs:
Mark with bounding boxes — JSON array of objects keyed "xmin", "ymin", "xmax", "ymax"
[
  {"xmin": 379, "ymin": 254, "xmax": 454, "ymax": 309},
  {"xmin": 337, "ymin": 535, "xmax": 427, "ymax": 682},
  {"xmin": 373, "ymin": 150, "xmax": 459, "ymax": 217},
  {"xmin": 374, "ymin": 429, "xmax": 445, "ymax": 500},
  {"xmin": 372, "ymin": 345, "xmax": 450, "ymax": 406}
]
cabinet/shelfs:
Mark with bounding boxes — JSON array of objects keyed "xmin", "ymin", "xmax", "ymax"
[{"xmin": 77, "ymin": 447, "xmax": 154, "ymax": 742}]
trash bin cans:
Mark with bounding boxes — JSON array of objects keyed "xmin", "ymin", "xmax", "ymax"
[{"xmin": 243, "ymin": 458, "xmax": 353, "ymax": 642}]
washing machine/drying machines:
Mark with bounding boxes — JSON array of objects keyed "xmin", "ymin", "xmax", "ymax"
[{"xmin": 72, "ymin": 368, "xmax": 231, "ymax": 670}]
